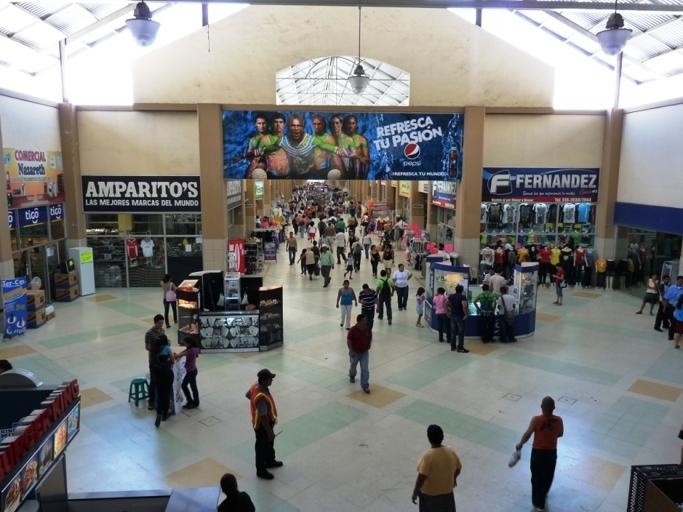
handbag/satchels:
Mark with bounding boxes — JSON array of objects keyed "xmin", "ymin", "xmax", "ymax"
[
  {"xmin": 504, "ymin": 309, "xmax": 515, "ymax": 321},
  {"xmin": 507, "ymin": 446, "xmax": 521, "ymax": 468},
  {"xmin": 658, "ymin": 287, "xmax": 662, "ymax": 301},
  {"xmin": 559, "ymin": 277, "xmax": 567, "ymax": 288},
  {"xmin": 351, "ymin": 288, "xmax": 355, "ymax": 300},
  {"xmin": 165, "ymin": 281, "xmax": 177, "ymax": 303}
]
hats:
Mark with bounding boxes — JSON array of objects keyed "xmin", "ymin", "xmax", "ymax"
[{"xmin": 257, "ymin": 368, "xmax": 276, "ymax": 379}]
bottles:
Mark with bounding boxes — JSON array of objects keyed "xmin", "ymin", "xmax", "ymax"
[{"xmin": 448, "ymin": 145, "xmax": 458, "ymax": 179}]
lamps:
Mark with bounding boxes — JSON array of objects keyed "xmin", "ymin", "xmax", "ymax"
[
  {"xmin": 596, "ymin": 1, "xmax": 634, "ymax": 57},
  {"xmin": 125, "ymin": 0, "xmax": 160, "ymax": 47},
  {"xmin": 348, "ymin": 5, "xmax": 370, "ymax": 96}
]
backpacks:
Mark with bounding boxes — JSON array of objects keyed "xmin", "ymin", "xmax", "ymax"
[
  {"xmin": 151, "ymin": 349, "xmax": 168, "ymax": 388},
  {"xmin": 479, "ymin": 291, "xmax": 493, "ymax": 320},
  {"xmin": 379, "ymin": 277, "xmax": 391, "ymax": 303}
]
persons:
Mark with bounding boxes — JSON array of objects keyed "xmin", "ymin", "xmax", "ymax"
[
  {"xmin": 345, "ymin": 313, "xmax": 374, "ymax": 395},
  {"xmin": 479, "ymin": 238, "xmax": 657, "ymax": 293},
  {"xmin": 148, "ymin": 333, "xmax": 174, "ymax": 428},
  {"xmin": 411, "ymin": 424, "xmax": 460, "ymax": 511},
  {"xmin": 245, "ymin": 368, "xmax": 283, "ymax": 481},
  {"xmin": 172, "ymin": 334, "xmax": 202, "ymax": 409},
  {"xmin": 414, "ymin": 263, "xmax": 518, "ymax": 353},
  {"xmin": 252, "ymin": 183, "xmax": 411, "ymax": 330},
  {"xmin": 215, "ymin": 473, "xmax": 256, "ymax": 511},
  {"xmin": 514, "ymin": 396, "xmax": 565, "ymax": 511},
  {"xmin": 159, "ymin": 272, "xmax": 178, "ymax": 329},
  {"xmin": 242, "ymin": 112, "xmax": 376, "ymax": 179},
  {"xmin": 634, "ymin": 273, "xmax": 683, "ymax": 349},
  {"xmin": 553, "ymin": 263, "xmax": 566, "ymax": 306},
  {"xmin": 144, "ymin": 314, "xmax": 165, "ymax": 410}
]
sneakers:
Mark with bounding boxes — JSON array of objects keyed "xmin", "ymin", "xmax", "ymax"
[
  {"xmin": 265, "ymin": 459, "xmax": 284, "ymax": 468},
  {"xmin": 668, "ymin": 336, "xmax": 680, "ymax": 349},
  {"xmin": 377, "ymin": 315, "xmax": 383, "ymax": 320},
  {"xmin": 635, "ymin": 310, "xmax": 642, "ymax": 314},
  {"xmin": 551, "ymin": 300, "xmax": 562, "ymax": 306},
  {"xmin": 450, "ymin": 345, "xmax": 470, "ymax": 353},
  {"xmin": 340, "ymin": 323, "xmax": 351, "ymax": 330},
  {"xmin": 364, "ymin": 388, "xmax": 370, "ymax": 393},
  {"xmin": 256, "ymin": 464, "xmax": 274, "ymax": 479},
  {"xmin": 398, "ymin": 305, "xmax": 406, "ymax": 311},
  {"xmin": 388, "ymin": 319, "xmax": 392, "ymax": 325},
  {"xmin": 481, "ymin": 336, "xmax": 517, "ymax": 344},
  {"xmin": 349, "ymin": 375, "xmax": 355, "ymax": 383},
  {"xmin": 416, "ymin": 322, "xmax": 424, "ymax": 328}
]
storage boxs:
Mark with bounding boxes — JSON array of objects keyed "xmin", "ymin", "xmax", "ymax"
[
  {"xmin": 53, "ymin": 270, "xmax": 79, "ymax": 302},
  {"xmin": 28, "ymin": 289, "xmax": 55, "ymax": 328}
]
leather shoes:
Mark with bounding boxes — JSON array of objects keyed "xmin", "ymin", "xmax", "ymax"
[
  {"xmin": 155, "ymin": 411, "xmax": 171, "ymax": 428},
  {"xmin": 654, "ymin": 325, "xmax": 671, "ymax": 332}
]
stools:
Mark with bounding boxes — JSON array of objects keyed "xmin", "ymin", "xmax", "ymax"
[{"xmin": 128, "ymin": 378, "xmax": 149, "ymax": 407}]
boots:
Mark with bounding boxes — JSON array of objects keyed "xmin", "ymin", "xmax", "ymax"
[{"xmin": 182, "ymin": 395, "xmax": 200, "ymax": 409}]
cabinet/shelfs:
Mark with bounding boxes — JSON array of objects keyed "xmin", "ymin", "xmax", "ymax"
[{"xmin": 243, "ymin": 228, "xmax": 277, "ymax": 275}]
